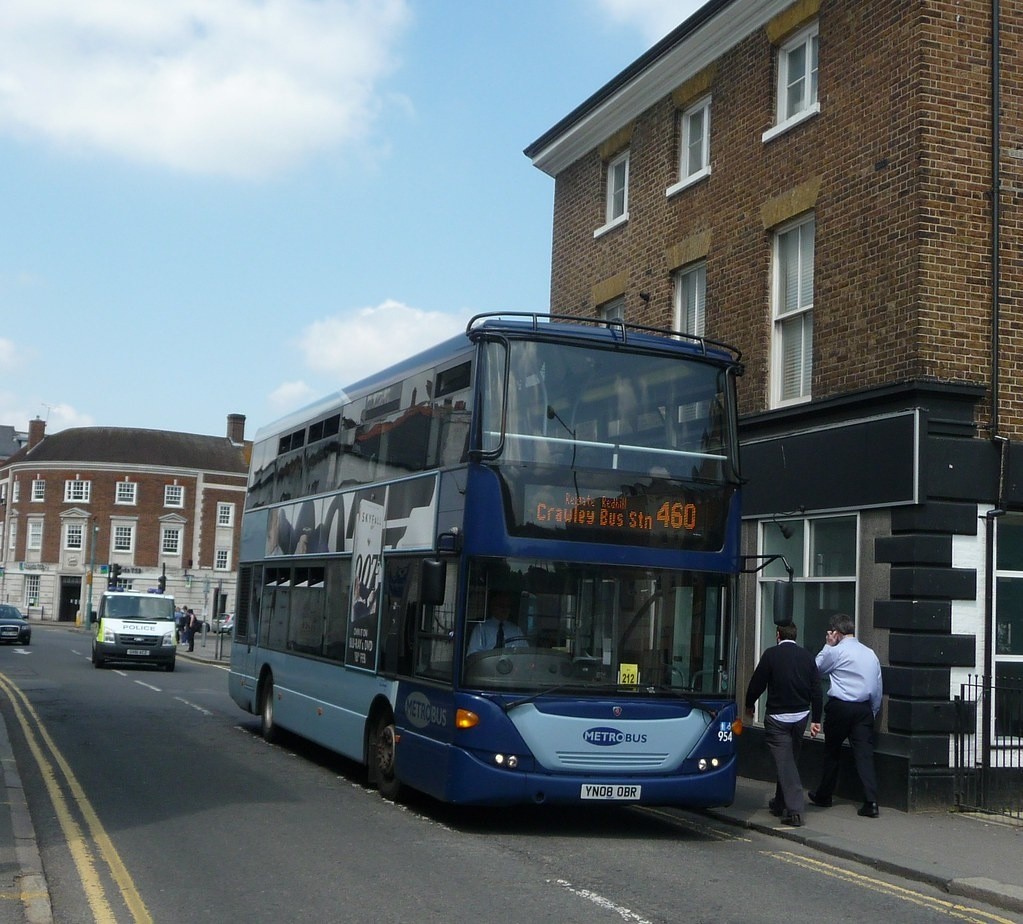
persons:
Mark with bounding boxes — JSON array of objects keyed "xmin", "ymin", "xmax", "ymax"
[
  {"xmin": 353, "ymin": 577, "xmax": 374, "ymax": 608},
  {"xmin": 744, "ymin": 623, "xmax": 822, "ymax": 828},
  {"xmin": 466, "ymin": 594, "xmax": 529, "ymax": 658},
  {"xmin": 174, "ymin": 606, "xmax": 183, "ymax": 644},
  {"xmin": 181, "ymin": 606, "xmax": 189, "ymax": 645},
  {"xmin": 266, "ymin": 507, "xmax": 307, "ymax": 556},
  {"xmin": 808, "ymin": 613, "xmax": 884, "ymax": 817},
  {"xmin": 185, "ymin": 609, "xmax": 196, "ymax": 652}
]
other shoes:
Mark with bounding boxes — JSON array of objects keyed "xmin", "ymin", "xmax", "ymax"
[
  {"xmin": 769, "ymin": 797, "xmax": 786, "ymax": 812},
  {"xmin": 782, "ymin": 815, "xmax": 801, "ymax": 826}
]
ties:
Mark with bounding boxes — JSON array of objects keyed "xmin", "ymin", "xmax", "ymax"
[{"xmin": 493, "ymin": 623, "xmax": 506, "ymax": 649}]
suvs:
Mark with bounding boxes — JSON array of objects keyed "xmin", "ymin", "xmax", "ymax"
[{"xmin": 89, "ymin": 590, "xmax": 178, "ymax": 671}]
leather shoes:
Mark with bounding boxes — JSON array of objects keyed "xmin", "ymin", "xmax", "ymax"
[
  {"xmin": 808, "ymin": 791, "xmax": 832, "ymax": 805},
  {"xmin": 858, "ymin": 802, "xmax": 880, "ymax": 817}
]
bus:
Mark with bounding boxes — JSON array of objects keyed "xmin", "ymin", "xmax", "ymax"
[{"xmin": 229, "ymin": 311, "xmax": 797, "ymax": 812}]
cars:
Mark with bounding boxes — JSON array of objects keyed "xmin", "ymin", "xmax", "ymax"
[
  {"xmin": 212, "ymin": 612, "xmax": 235, "ymax": 633},
  {"xmin": 0, "ymin": 604, "xmax": 31, "ymax": 644}
]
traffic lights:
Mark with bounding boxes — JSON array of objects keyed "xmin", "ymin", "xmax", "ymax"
[
  {"xmin": 158, "ymin": 576, "xmax": 166, "ymax": 590},
  {"xmin": 113, "ymin": 564, "xmax": 121, "ymax": 582}
]
